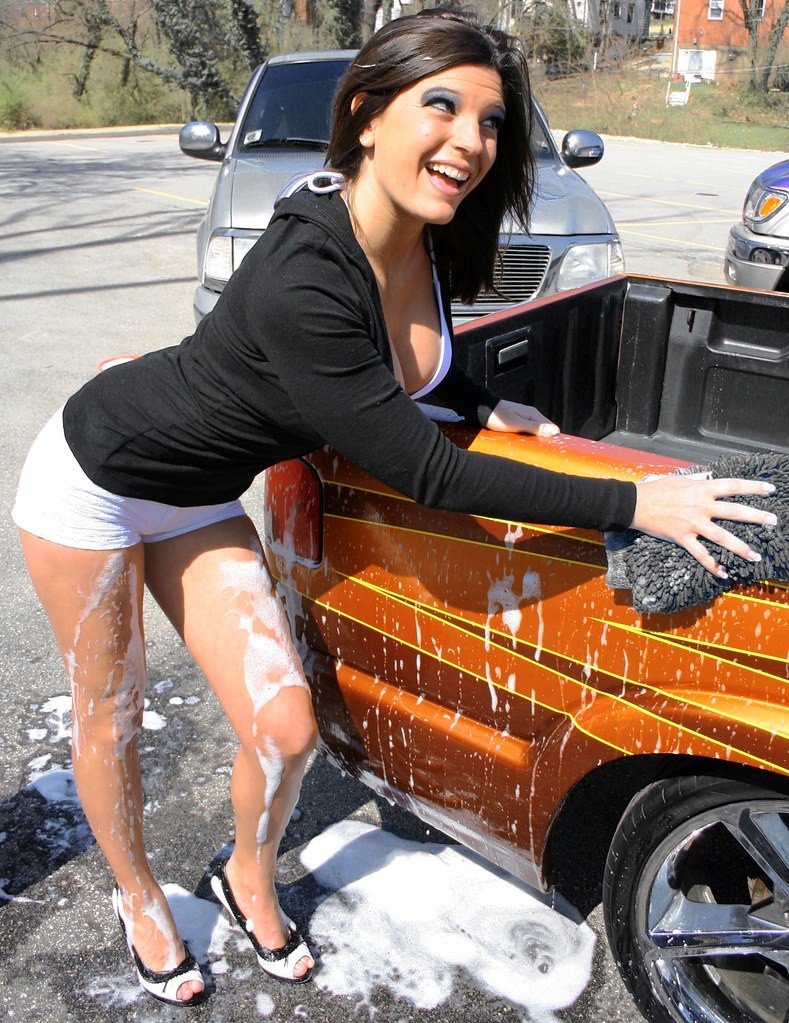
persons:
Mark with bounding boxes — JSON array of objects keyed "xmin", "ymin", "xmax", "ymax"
[{"xmin": 12, "ymin": 12, "xmax": 780, "ymax": 1004}]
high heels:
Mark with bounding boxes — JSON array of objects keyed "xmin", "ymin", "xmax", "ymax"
[
  {"xmin": 209, "ymin": 862, "xmax": 319, "ymax": 987},
  {"xmin": 112, "ymin": 885, "xmax": 206, "ymax": 1002}
]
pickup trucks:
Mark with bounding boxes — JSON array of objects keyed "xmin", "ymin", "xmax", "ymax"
[{"xmin": 262, "ymin": 271, "xmax": 789, "ymax": 1022}]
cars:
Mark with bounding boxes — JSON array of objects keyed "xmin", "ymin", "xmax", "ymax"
[{"xmin": 179, "ymin": 45, "xmax": 624, "ymax": 325}]
